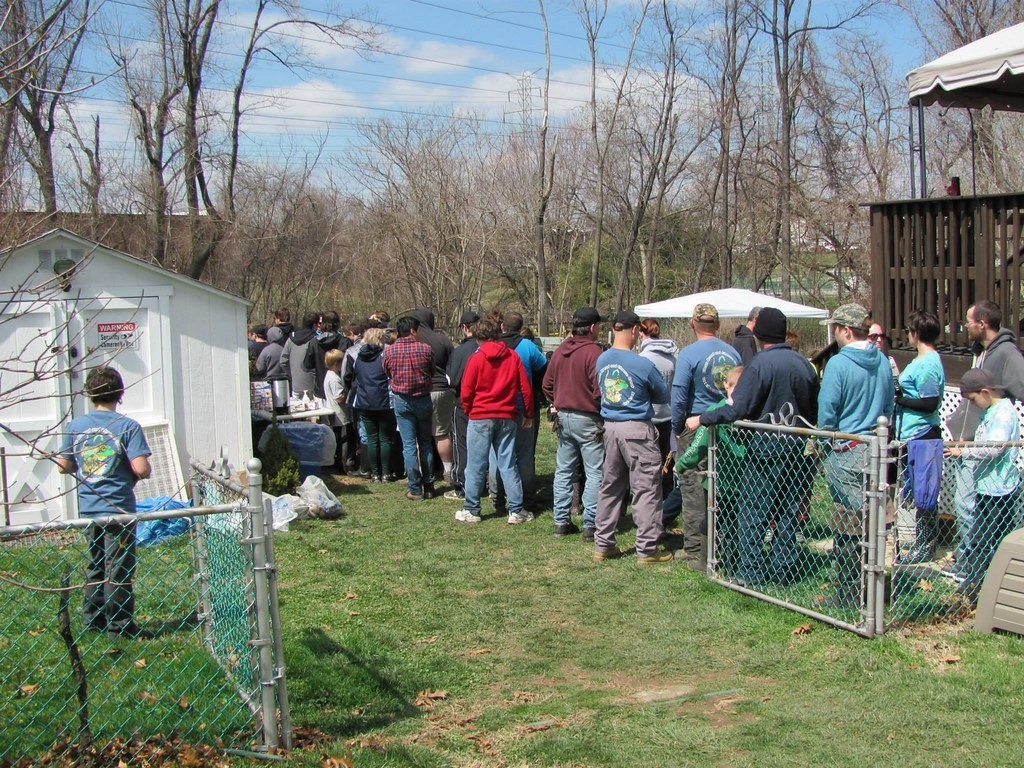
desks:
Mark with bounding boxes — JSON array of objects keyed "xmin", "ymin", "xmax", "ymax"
[{"xmin": 252, "ymin": 409, "xmax": 335, "ymax": 423}]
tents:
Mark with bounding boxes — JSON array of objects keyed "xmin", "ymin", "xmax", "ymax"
[{"xmin": 635, "ymin": 288, "xmax": 830, "ymax": 342}]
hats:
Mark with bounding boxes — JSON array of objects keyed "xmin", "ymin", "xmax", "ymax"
[
  {"xmin": 253, "ymin": 324, "xmax": 266, "ymax": 334},
  {"xmin": 960, "ymin": 368, "xmax": 1007, "ymax": 394},
  {"xmin": 612, "ymin": 312, "xmax": 647, "ymax": 331},
  {"xmin": 819, "ymin": 304, "xmax": 872, "ymax": 330},
  {"xmin": 572, "ymin": 307, "xmax": 608, "ymax": 327},
  {"xmin": 753, "ymin": 307, "xmax": 787, "ymax": 343},
  {"xmin": 456, "ymin": 311, "xmax": 480, "ymax": 328},
  {"xmin": 692, "ymin": 303, "xmax": 719, "ymax": 323}
]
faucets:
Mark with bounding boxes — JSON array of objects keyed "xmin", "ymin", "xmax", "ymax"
[{"xmin": 283, "ymin": 401, "xmax": 288, "ymax": 407}]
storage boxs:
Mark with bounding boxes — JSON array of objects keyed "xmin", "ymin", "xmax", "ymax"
[{"xmin": 250, "ymin": 381, "xmax": 274, "ymax": 412}]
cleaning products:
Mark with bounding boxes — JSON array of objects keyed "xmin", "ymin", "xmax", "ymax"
[{"xmin": 302, "ymin": 389, "xmax": 310, "ymax": 411}]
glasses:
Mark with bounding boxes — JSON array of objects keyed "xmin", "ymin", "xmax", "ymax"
[{"xmin": 868, "ymin": 333, "xmax": 885, "ymax": 341}]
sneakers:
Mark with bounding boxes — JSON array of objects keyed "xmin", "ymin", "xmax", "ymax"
[
  {"xmin": 455, "ymin": 509, "xmax": 481, "ymax": 522},
  {"xmin": 508, "ymin": 509, "xmax": 534, "ymax": 524}
]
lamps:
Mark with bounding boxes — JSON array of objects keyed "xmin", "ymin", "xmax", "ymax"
[{"xmin": 53, "ymin": 257, "xmax": 76, "ymax": 292}]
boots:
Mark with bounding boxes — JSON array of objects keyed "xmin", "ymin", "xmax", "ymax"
[
  {"xmin": 686, "ymin": 534, "xmax": 707, "ymax": 572},
  {"xmin": 900, "ymin": 511, "xmax": 936, "ymax": 564}
]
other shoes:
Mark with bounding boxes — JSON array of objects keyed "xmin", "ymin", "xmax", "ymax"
[
  {"xmin": 346, "ymin": 465, "xmax": 371, "ymax": 479},
  {"xmin": 594, "ymin": 547, "xmax": 620, "ymax": 559},
  {"xmin": 673, "ymin": 549, "xmax": 700, "ymax": 561},
  {"xmin": 637, "ymin": 551, "xmax": 673, "ymax": 563},
  {"xmin": 108, "ymin": 622, "xmax": 151, "ymax": 639},
  {"xmin": 444, "ymin": 489, "xmax": 465, "ymax": 500},
  {"xmin": 405, "ymin": 490, "xmax": 438, "ymax": 500},
  {"xmin": 727, "ymin": 565, "xmax": 767, "ymax": 586},
  {"xmin": 555, "ymin": 523, "xmax": 579, "ymax": 535},
  {"xmin": 769, "ymin": 562, "xmax": 794, "ymax": 585},
  {"xmin": 371, "ymin": 470, "xmax": 392, "ymax": 484},
  {"xmin": 941, "ymin": 562, "xmax": 970, "ymax": 582},
  {"xmin": 583, "ymin": 528, "xmax": 596, "ymax": 541}
]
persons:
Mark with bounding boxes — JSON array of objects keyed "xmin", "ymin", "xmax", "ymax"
[
  {"xmin": 671, "ymin": 303, "xmax": 743, "ymax": 552},
  {"xmin": 455, "ymin": 318, "xmax": 535, "ymax": 525},
  {"xmin": 381, "ymin": 316, "xmax": 436, "ymax": 502},
  {"xmin": 786, "ymin": 332, "xmax": 819, "ymax": 376},
  {"xmin": 356, "ymin": 328, "xmax": 396, "ymax": 482},
  {"xmin": 686, "ymin": 307, "xmax": 820, "ymax": 585},
  {"xmin": 867, "ymin": 322, "xmax": 900, "ymax": 397},
  {"xmin": 894, "ymin": 307, "xmax": 945, "ymax": 564},
  {"xmin": 542, "ymin": 307, "xmax": 605, "ymax": 542},
  {"xmin": 958, "ymin": 368, "xmax": 1019, "ymax": 595},
  {"xmin": 733, "ymin": 306, "xmax": 764, "ymax": 365},
  {"xmin": 816, "ymin": 303, "xmax": 893, "ymax": 607},
  {"xmin": 638, "ymin": 317, "xmax": 681, "ymax": 503},
  {"xmin": 594, "ymin": 312, "xmax": 673, "ymax": 562},
  {"xmin": 940, "ymin": 300, "xmax": 1024, "ymax": 581},
  {"xmin": 248, "ymin": 306, "xmax": 539, "ymax": 501},
  {"xmin": 57, "ymin": 367, "xmax": 151, "ymax": 632},
  {"xmin": 673, "ymin": 365, "xmax": 745, "ymax": 559}
]
